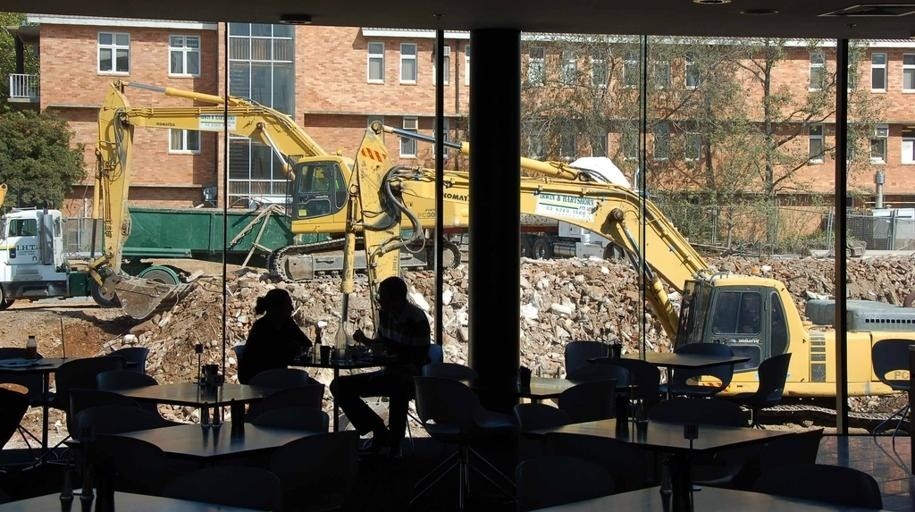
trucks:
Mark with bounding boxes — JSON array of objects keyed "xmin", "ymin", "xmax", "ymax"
[
  {"xmin": 0, "ymin": 197, "xmax": 181, "ymax": 315},
  {"xmin": 521, "ymin": 179, "xmax": 653, "ymax": 264}
]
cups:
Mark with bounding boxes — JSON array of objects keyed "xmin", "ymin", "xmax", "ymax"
[
  {"xmin": 612, "ymin": 344, "xmax": 622, "ymax": 360},
  {"xmin": 320, "ymin": 345, "xmax": 330, "ymax": 362}
]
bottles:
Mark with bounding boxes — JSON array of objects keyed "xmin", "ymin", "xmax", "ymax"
[
  {"xmin": 613, "ymin": 393, "xmax": 649, "ymax": 433},
  {"xmin": 26, "ymin": 335, "xmax": 37, "ymax": 361},
  {"xmin": 607, "ymin": 344, "xmax": 613, "ymax": 358},
  {"xmin": 60, "ymin": 461, "xmax": 94, "ymax": 501},
  {"xmin": 331, "ymin": 345, "xmax": 336, "ymax": 361},
  {"xmin": 662, "ymin": 455, "xmax": 694, "ymax": 494},
  {"xmin": 334, "ymin": 319, "xmax": 347, "ymax": 361},
  {"xmin": 200, "ymin": 364, "xmax": 222, "ymax": 388},
  {"xmin": 199, "ymin": 402, "xmax": 221, "ymax": 427},
  {"xmin": 313, "ymin": 328, "xmax": 323, "ymax": 359}
]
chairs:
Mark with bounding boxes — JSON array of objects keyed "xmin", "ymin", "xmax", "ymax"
[{"xmin": 872, "ymin": 339, "xmax": 915, "ymax": 445}]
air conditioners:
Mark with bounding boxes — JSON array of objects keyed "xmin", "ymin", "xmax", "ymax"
[
  {"xmin": 874, "ymin": 127, "xmax": 888, "ymax": 138},
  {"xmin": 402, "ymin": 117, "xmax": 419, "ymax": 130},
  {"xmin": 443, "ymin": 46, "xmax": 450, "ymax": 56}
]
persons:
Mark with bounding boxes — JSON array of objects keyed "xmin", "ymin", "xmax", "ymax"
[
  {"xmin": 330, "ymin": 277, "xmax": 430, "ymax": 459},
  {"xmin": 239, "ymin": 289, "xmax": 319, "ymax": 386}
]
